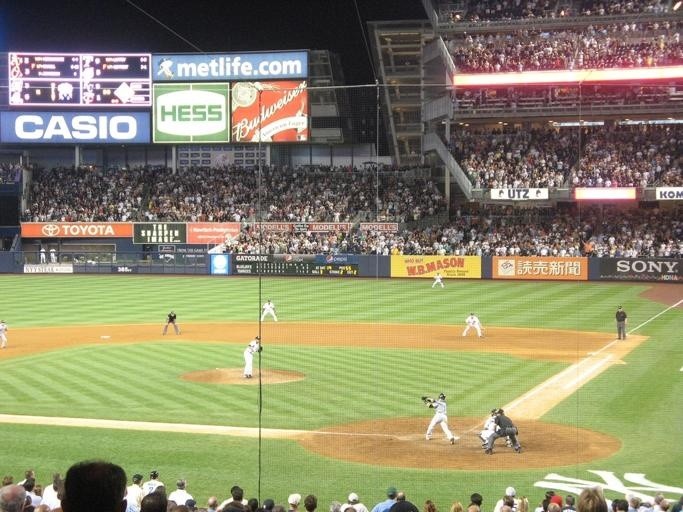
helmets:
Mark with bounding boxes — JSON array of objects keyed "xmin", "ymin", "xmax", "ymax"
[
  {"xmin": 150, "ymin": 471, "xmax": 159, "ymax": 479},
  {"xmin": 439, "ymin": 393, "xmax": 446, "ymax": 400},
  {"xmin": 495, "ymin": 409, "xmax": 504, "ymax": 415}
]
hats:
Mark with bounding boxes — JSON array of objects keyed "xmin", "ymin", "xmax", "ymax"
[
  {"xmin": 186, "ymin": 499, "xmax": 197, "ymax": 507},
  {"xmin": 133, "ymin": 473, "xmax": 143, "ymax": 483},
  {"xmin": 262, "ymin": 499, "xmax": 275, "ymax": 509},
  {"xmin": 288, "ymin": 493, "xmax": 301, "ymax": 506},
  {"xmin": 506, "ymin": 486, "xmax": 516, "ymax": 497},
  {"xmin": 386, "ymin": 487, "xmax": 396, "ymax": 497},
  {"xmin": 348, "ymin": 493, "xmax": 358, "ymax": 502},
  {"xmin": 176, "ymin": 479, "xmax": 185, "ymax": 488}
]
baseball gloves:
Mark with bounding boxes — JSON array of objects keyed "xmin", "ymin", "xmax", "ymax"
[{"xmin": 258, "ymin": 347, "xmax": 262, "ymax": 352}]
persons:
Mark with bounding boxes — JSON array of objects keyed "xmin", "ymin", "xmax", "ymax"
[
  {"xmin": 462, "ymin": 313, "xmax": 482, "ymax": 337},
  {"xmin": 260, "ymin": 298, "xmax": 278, "ymax": 322},
  {"xmin": 486, "ymin": 409, "xmax": 521, "ymax": 455},
  {"xmin": 422, "ymin": 393, "xmax": 457, "ymax": 444},
  {"xmin": 242, "ymin": 336, "xmax": 263, "ymax": 378},
  {"xmin": 162, "ymin": 311, "xmax": 181, "ymax": 336},
  {"xmin": 0, "ymin": 319, "xmax": 9, "ymax": 349},
  {"xmin": 615, "ymin": 305, "xmax": 627, "ymax": 340},
  {"xmin": 432, "ymin": 272, "xmax": 445, "ymax": 289},
  {"xmin": 479, "ymin": 409, "xmax": 512, "ymax": 449}
]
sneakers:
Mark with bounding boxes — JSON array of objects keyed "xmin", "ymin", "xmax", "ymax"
[
  {"xmin": 485, "ymin": 449, "xmax": 493, "ymax": 455},
  {"xmin": 449, "ymin": 436, "xmax": 455, "ymax": 445},
  {"xmin": 504, "ymin": 442, "xmax": 512, "ymax": 447}
]
